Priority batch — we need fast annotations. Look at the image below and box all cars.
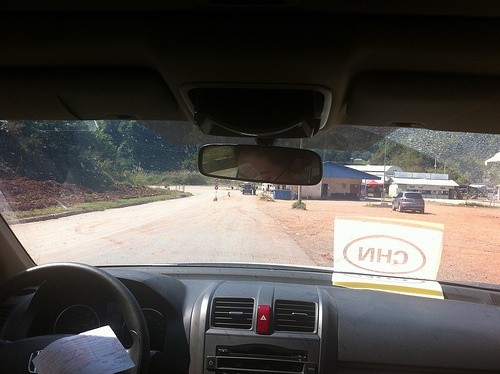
[391,191,424,214]
[242,185,252,195]
